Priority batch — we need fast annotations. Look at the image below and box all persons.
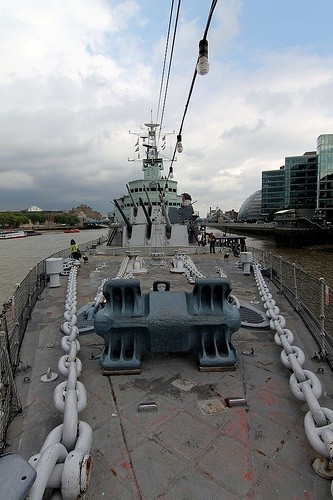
[197,233,216,254]
[70,238,80,261]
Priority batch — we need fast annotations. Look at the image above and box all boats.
[64,229,80,233]
[23,230,42,237]
[0,109,333,500]
[0,231,27,239]
